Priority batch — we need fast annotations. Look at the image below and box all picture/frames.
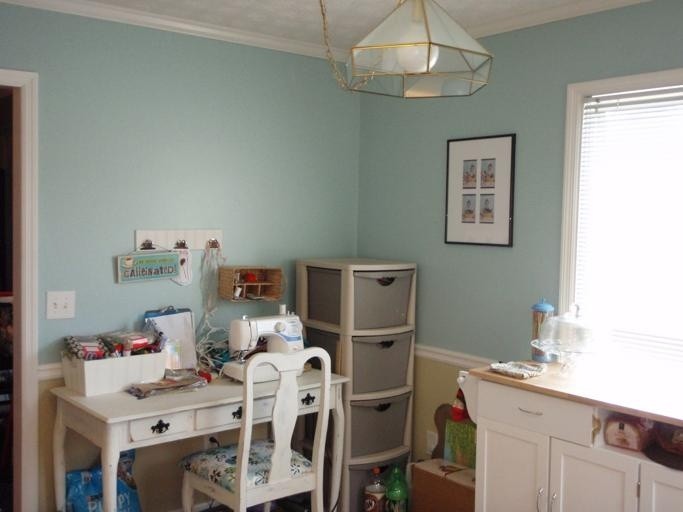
[444,133,518,247]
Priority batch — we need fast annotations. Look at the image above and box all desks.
[50,375,352,510]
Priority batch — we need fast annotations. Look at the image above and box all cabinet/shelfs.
[218,264,282,302]
[293,253,419,510]
[469,358,682,512]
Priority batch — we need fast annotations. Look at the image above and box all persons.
[464,164,476,183]
[481,199,492,217]
[483,163,494,184]
[463,200,475,218]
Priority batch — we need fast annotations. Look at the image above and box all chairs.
[178,345,333,512]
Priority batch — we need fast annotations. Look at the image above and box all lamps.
[339,0,495,100]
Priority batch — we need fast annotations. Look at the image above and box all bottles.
[362,467,386,511]
[386,467,409,511]
[530,297,554,362]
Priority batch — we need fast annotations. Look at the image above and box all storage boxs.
[60,348,165,397]
